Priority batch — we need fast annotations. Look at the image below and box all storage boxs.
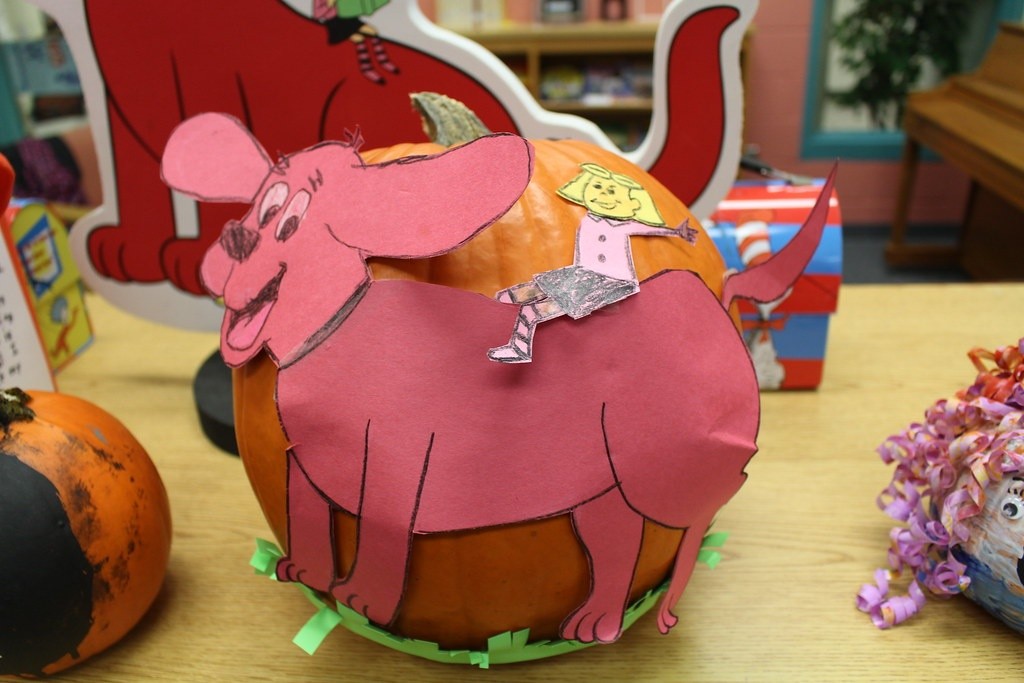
[0,191,54,394]
[702,178,842,393]
[11,198,95,374]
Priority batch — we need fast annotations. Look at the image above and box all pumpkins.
[233,90,744,652]
[0,387,173,679]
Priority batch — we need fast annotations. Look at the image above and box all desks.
[0,282,1024,682]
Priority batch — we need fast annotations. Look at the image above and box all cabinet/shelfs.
[467,27,753,155]
